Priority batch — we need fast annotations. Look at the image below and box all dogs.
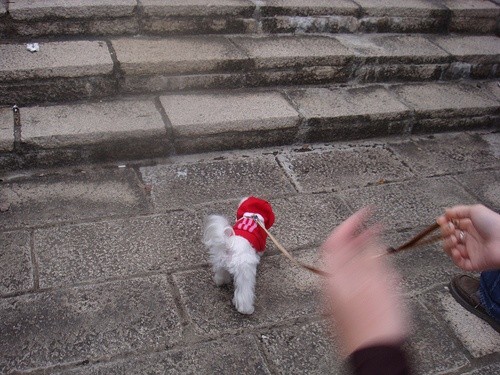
[201,196,275,315]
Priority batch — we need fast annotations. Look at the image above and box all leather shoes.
[449,273,500,335]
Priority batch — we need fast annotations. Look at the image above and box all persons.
[321,203,500,375]
[449,269,500,334]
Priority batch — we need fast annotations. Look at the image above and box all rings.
[459,229,465,243]
